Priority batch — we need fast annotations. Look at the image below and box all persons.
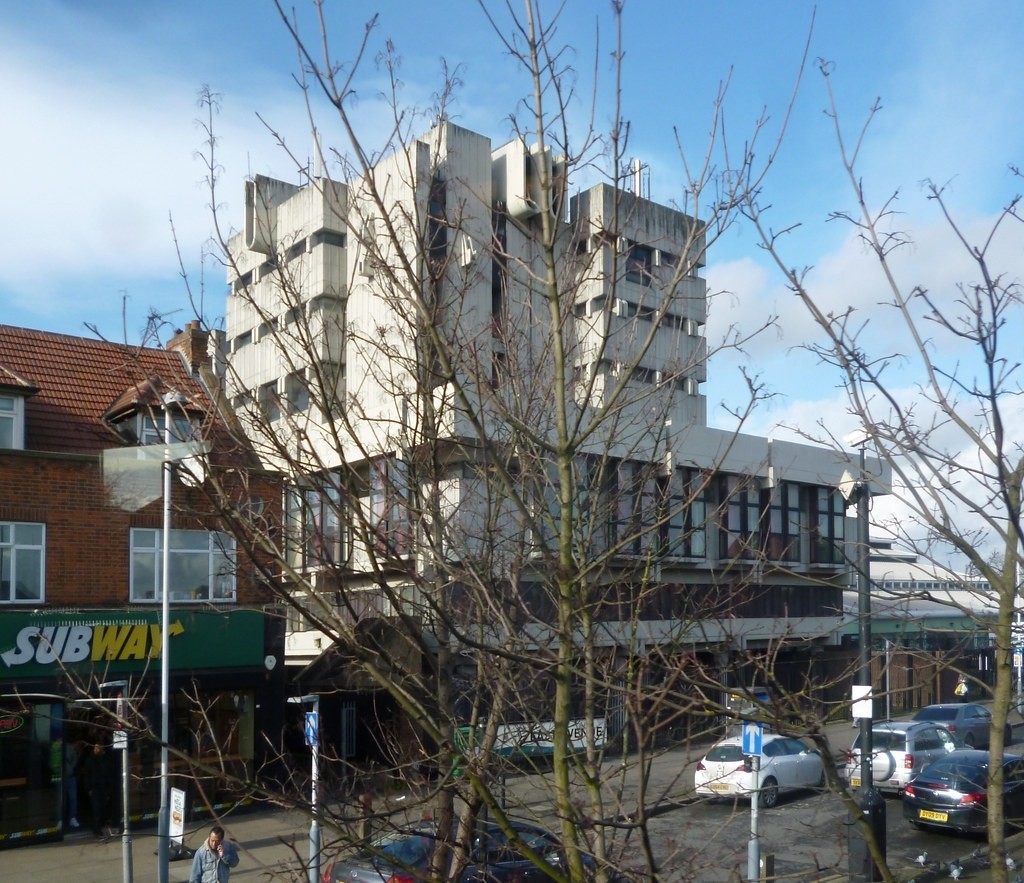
[189,826,239,883]
[957,673,968,703]
[80,741,119,841]
[49,729,79,830]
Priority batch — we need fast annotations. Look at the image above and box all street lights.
[288,693,322,883]
[97,680,139,883]
[155,391,187,883]
[843,429,891,883]
[728,686,773,883]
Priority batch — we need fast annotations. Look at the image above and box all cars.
[912,703,1013,749]
[323,813,598,883]
[902,748,1024,835]
[693,733,832,810]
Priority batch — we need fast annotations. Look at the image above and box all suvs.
[844,719,976,796]
